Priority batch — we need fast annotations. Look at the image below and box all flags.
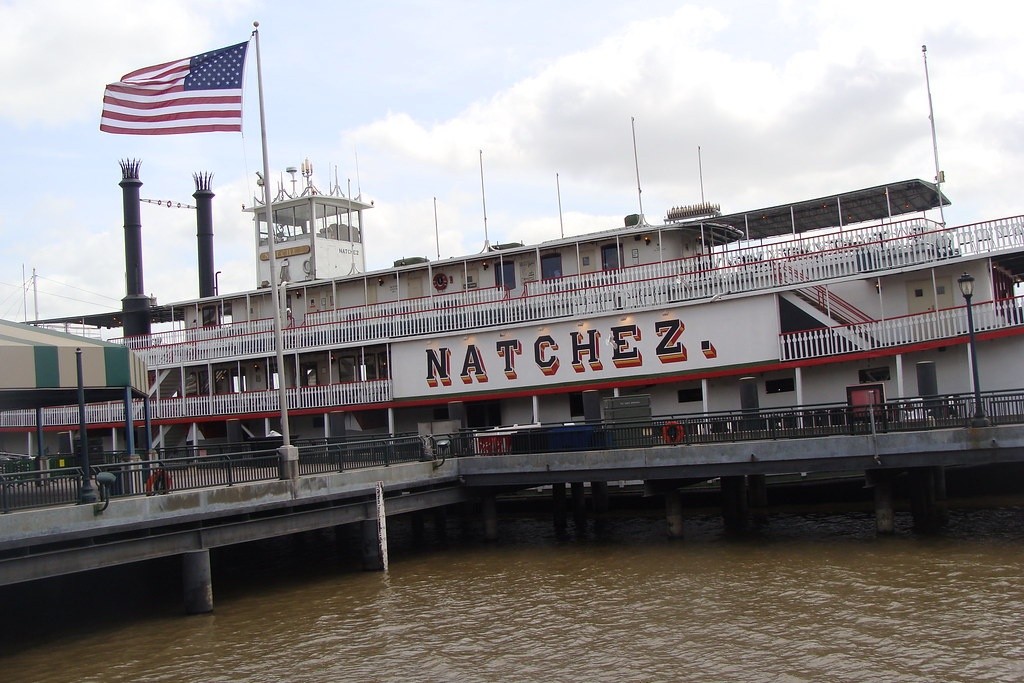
[99,40,250,136]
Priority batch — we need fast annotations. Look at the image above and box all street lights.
[957,272,990,428]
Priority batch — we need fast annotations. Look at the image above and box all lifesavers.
[433,273,449,291]
[147,472,173,496]
[663,420,685,446]
